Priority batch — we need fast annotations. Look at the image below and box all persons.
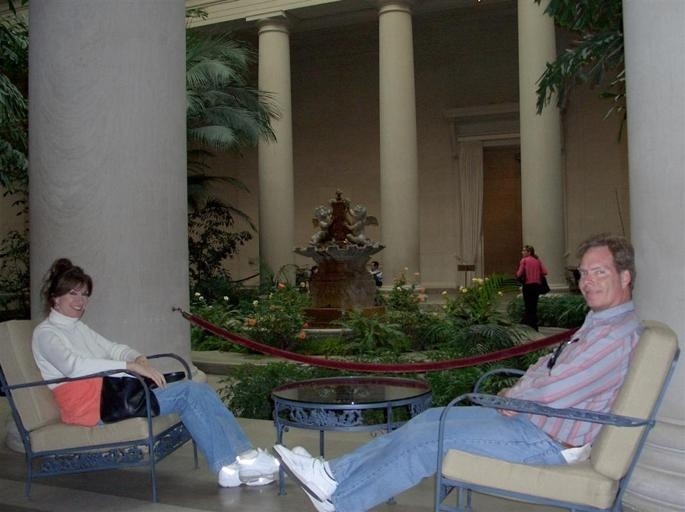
[271,231,640,511]
[29,257,288,488]
[369,260,383,287]
[513,244,548,331]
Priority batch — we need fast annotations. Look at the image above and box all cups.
[547,336,579,376]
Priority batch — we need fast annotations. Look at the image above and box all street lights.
[100,371,186,423]
[538,276,550,295]
[516,259,527,284]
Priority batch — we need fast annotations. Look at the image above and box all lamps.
[1,318,199,504]
[435,321,680,512]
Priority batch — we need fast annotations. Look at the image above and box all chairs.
[291,446,337,512]
[217,459,276,488]
[272,443,339,502]
[235,447,289,484]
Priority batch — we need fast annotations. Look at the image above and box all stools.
[544,432,573,448]
